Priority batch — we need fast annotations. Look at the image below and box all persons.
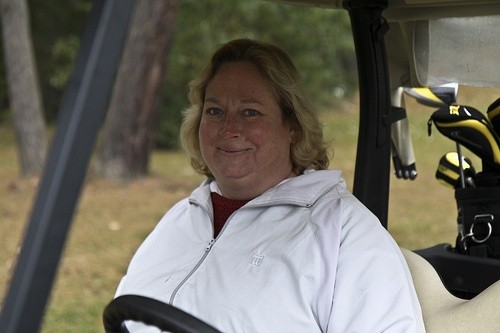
[110,39,429,333]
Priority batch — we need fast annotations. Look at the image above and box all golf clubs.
[439,82,468,188]
